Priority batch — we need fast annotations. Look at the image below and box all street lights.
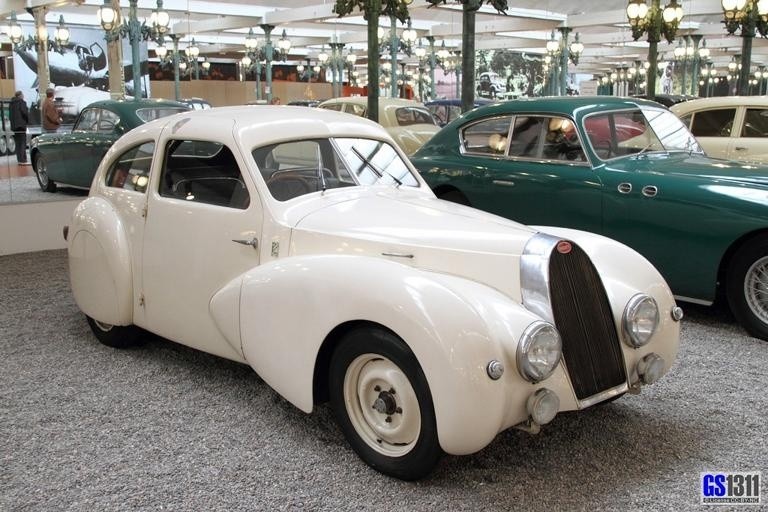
[8,9,69,106]
[184,37,199,79]
[245,0,767,105]
[97,0,169,98]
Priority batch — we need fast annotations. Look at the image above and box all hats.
[46,88,54,93]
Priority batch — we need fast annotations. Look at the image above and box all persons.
[40,88,63,134]
[8,91,31,165]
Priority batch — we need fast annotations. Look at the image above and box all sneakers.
[17,161,31,165]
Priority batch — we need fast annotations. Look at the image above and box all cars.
[63,103,685,482]
[424,99,494,128]
[563,92,700,149]
[616,93,767,165]
[407,94,767,342]
[315,95,443,158]
[30,98,193,192]
